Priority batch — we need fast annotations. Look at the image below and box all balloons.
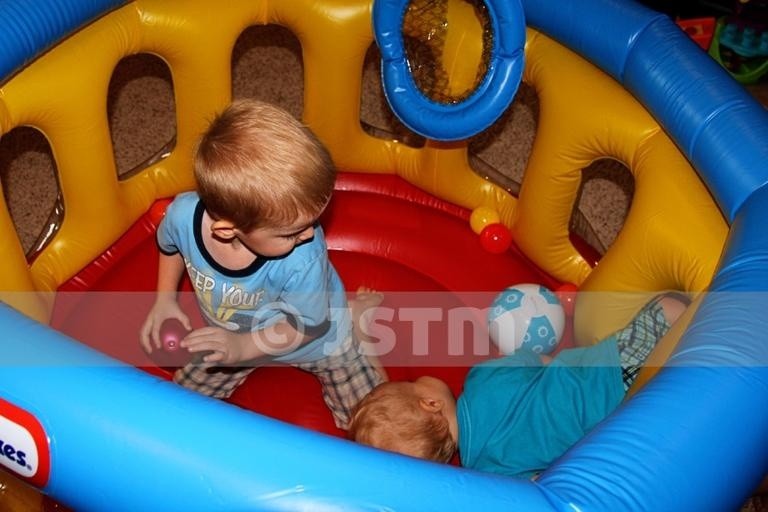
[477,222,512,255]
[469,204,500,235]
[144,319,196,369]
[555,282,579,316]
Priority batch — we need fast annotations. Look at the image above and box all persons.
[344,290,690,482]
[138,98,392,432]
